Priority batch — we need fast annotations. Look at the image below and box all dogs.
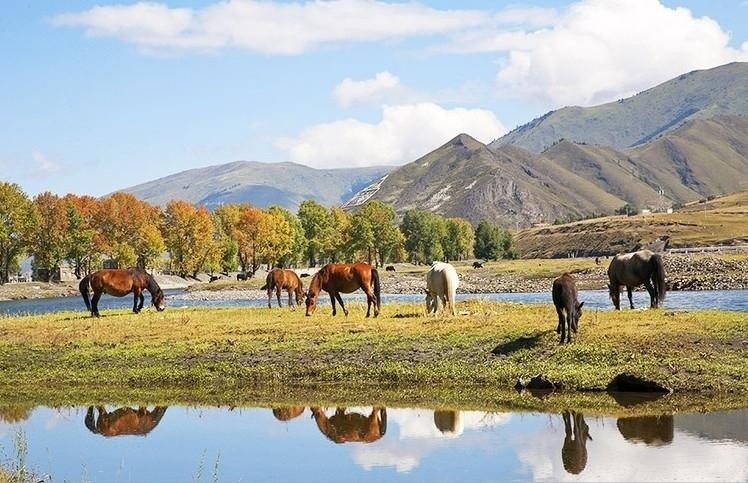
[561,410,594,475]
[305,262,381,318]
[78,266,165,318]
[606,249,667,311]
[552,272,584,345]
[259,269,306,310]
[423,261,459,316]
[309,405,387,445]
[84,405,169,438]
